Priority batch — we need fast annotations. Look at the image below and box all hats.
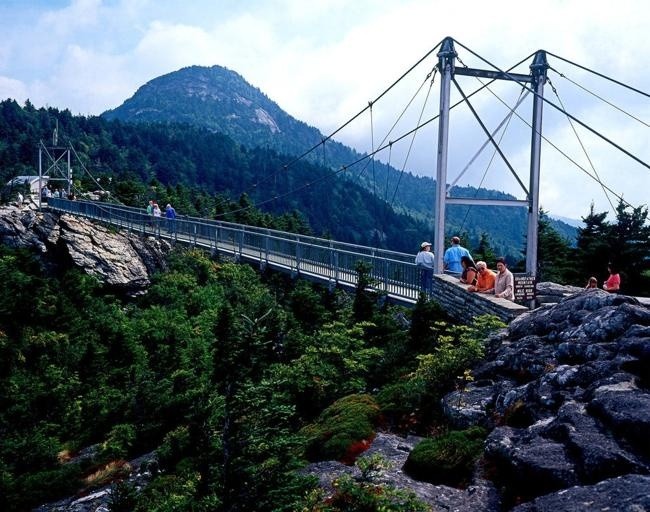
[421,241,432,248]
[451,236,460,243]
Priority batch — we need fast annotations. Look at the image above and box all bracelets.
[474,288,477,291]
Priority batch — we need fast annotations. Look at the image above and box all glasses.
[478,267,485,271]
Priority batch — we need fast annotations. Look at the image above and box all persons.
[596,260,621,293]
[414,242,434,299]
[459,256,479,287]
[616,270,632,295]
[16,191,24,209]
[147,200,161,235]
[443,237,476,280]
[467,260,496,292]
[165,204,178,234]
[480,259,515,302]
[583,275,598,289]
[42,184,77,201]
